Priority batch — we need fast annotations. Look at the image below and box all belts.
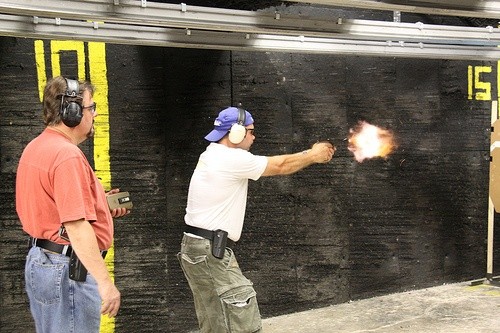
[33,238,107,261]
[184,224,235,251]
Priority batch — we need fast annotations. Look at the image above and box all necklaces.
[49,125,73,141]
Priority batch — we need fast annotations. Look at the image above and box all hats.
[204,107,254,142]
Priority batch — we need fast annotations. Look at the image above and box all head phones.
[61,79,83,127]
[229,107,247,145]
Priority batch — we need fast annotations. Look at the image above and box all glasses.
[83,102,96,112]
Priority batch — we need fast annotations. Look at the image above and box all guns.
[330,137,351,148]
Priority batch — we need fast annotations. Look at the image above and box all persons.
[16,75,132,333]
[177,107,338,333]
